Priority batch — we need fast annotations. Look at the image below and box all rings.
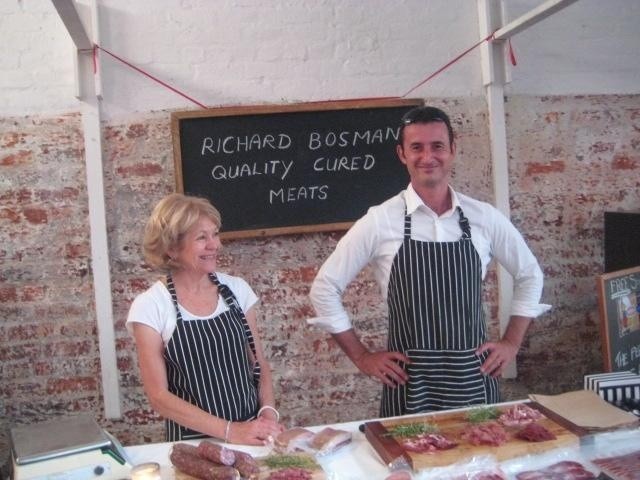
[268,435,274,443]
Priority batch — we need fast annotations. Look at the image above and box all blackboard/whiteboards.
[171,99,424,240]
[597,266,640,376]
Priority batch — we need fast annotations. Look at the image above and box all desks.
[122,388,639,479]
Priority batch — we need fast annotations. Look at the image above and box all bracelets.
[258,405,279,423]
[224,419,231,444]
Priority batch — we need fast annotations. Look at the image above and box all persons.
[308,107,544,419]
[126,193,288,446]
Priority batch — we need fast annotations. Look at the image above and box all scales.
[10,413,134,480]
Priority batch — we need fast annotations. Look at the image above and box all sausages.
[170,441,255,480]
[517,450,640,480]
